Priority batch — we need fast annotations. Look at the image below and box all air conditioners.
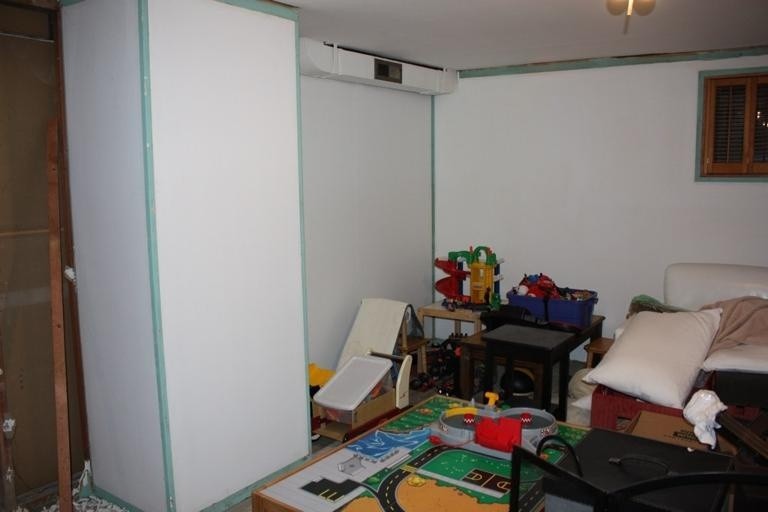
[299,37,458,96]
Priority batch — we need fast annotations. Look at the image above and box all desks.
[251,394,590,512]
[417,301,489,379]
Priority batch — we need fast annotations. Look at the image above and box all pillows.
[581,307,723,412]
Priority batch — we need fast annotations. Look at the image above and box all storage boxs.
[312,355,394,426]
[545,383,742,512]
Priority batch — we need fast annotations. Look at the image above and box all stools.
[480,323,575,422]
[583,337,614,368]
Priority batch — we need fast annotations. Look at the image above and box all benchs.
[614,262,768,397]
